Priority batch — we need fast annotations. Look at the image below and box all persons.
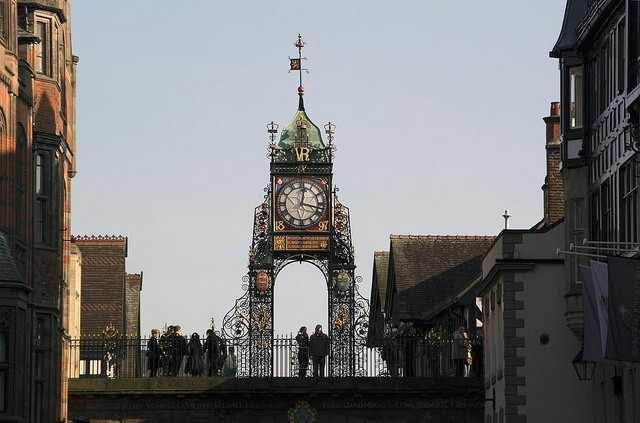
[463,328,473,377]
[309,324,331,377]
[145,325,239,378]
[452,326,465,375]
[297,326,310,376]
